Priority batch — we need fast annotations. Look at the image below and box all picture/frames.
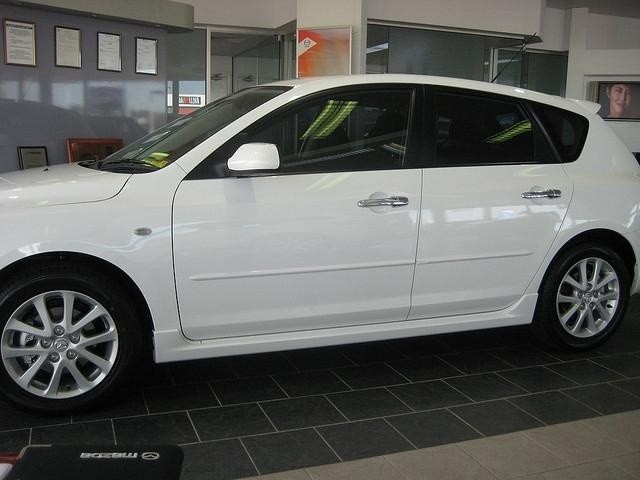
[17,138,124,170]
[3,17,159,76]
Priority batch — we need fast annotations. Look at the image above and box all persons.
[602,84,640,119]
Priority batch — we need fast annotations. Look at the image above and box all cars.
[0,74,640,413]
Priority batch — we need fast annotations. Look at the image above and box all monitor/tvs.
[594,80,640,122]
